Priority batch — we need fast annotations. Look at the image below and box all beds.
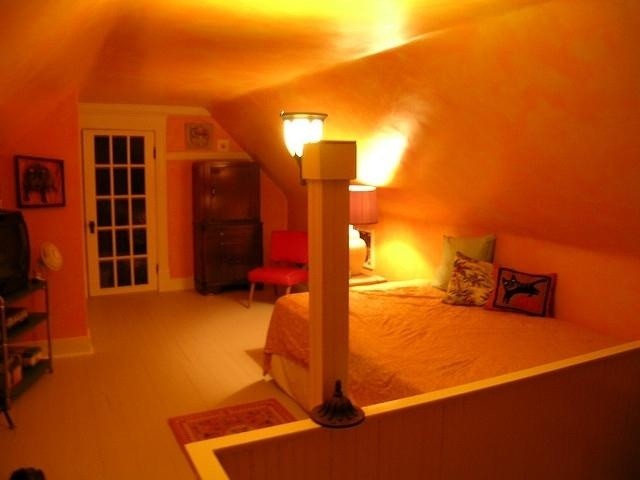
[264,231,640,423]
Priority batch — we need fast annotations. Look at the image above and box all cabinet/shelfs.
[0,279,53,421]
[193,158,262,294]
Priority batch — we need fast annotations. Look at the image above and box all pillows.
[432,233,560,317]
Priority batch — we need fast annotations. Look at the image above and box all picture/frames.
[183,121,213,149]
[15,153,66,210]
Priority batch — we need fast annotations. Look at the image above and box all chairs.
[246,229,308,309]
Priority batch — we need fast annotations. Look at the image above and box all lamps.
[279,111,328,187]
[346,185,379,270]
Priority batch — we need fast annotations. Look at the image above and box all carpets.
[168,397,299,480]
